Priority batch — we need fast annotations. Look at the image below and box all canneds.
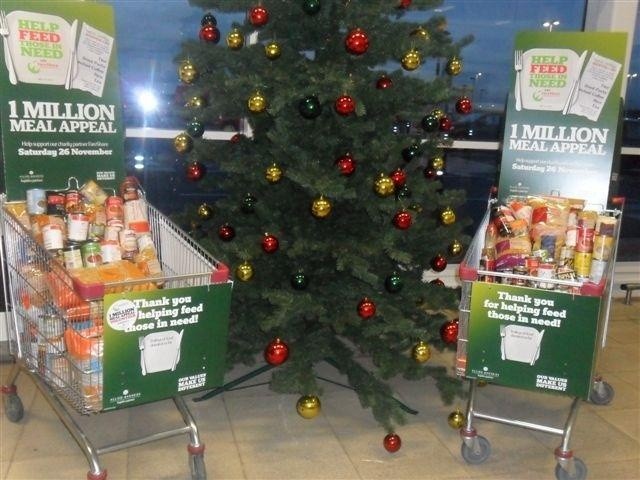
[121,176,138,200]
[79,179,109,206]
[572,214,614,284]
[41,189,101,270]
[119,229,139,261]
[480,259,496,283]
[104,195,124,228]
[26,188,46,214]
[30,314,70,388]
[513,249,555,289]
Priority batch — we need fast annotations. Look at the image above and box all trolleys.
[0,175,234,480]
[455,186,625,480]
